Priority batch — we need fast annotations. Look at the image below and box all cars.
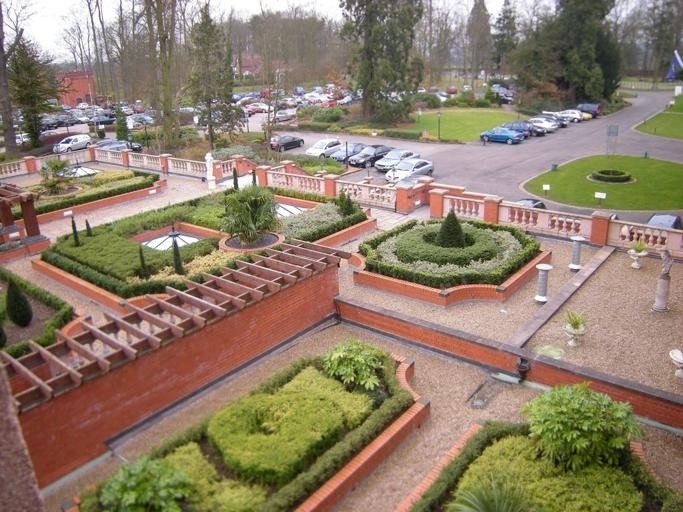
[13,131,58,145]
[0,83,471,130]
[52,133,142,153]
[645,212,681,229]
[305,138,434,182]
[270,135,304,151]
[480,83,601,145]
[513,198,547,210]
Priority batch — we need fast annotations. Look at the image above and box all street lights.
[450,47,461,78]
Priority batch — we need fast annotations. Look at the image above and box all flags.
[665,50,683,82]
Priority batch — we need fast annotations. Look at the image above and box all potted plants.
[626,237,649,270]
[563,309,589,348]
